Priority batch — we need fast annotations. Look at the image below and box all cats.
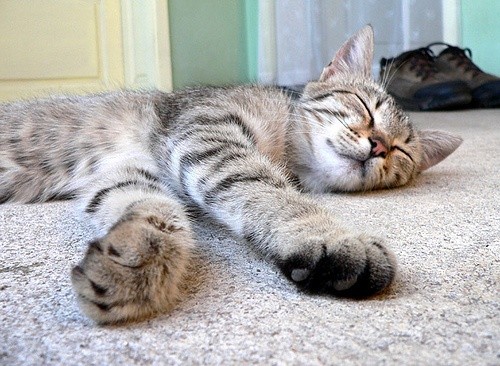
[0,21,465,329]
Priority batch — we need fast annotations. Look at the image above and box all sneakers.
[380,48,473,111]
[424,43,500,108]
